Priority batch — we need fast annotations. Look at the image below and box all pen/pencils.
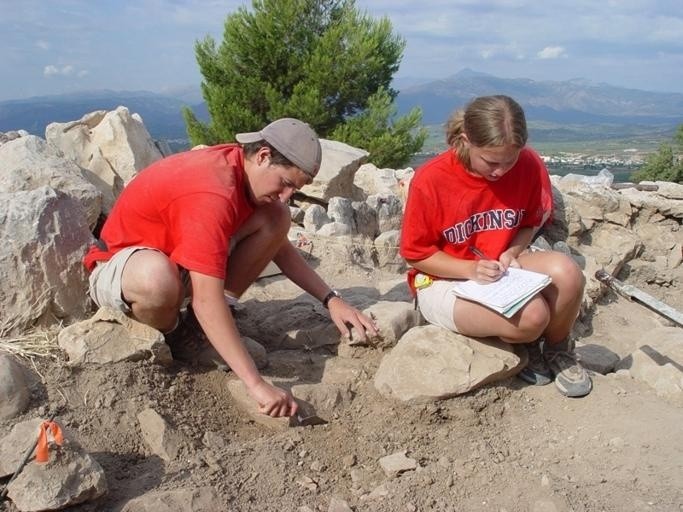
[470,246,488,260]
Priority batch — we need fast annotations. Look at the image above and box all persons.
[79,116,380,418]
[398,92,595,398]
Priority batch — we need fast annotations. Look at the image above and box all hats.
[235,116,322,177]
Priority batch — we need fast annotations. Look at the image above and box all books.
[451,266,553,320]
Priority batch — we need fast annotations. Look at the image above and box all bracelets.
[323,289,342,309]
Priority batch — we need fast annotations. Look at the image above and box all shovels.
[287,409,328,428]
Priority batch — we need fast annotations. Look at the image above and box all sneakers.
[186,302,268,371]
[541,331,593,397]
[516,339,552,387]
[162,310,232,371]
